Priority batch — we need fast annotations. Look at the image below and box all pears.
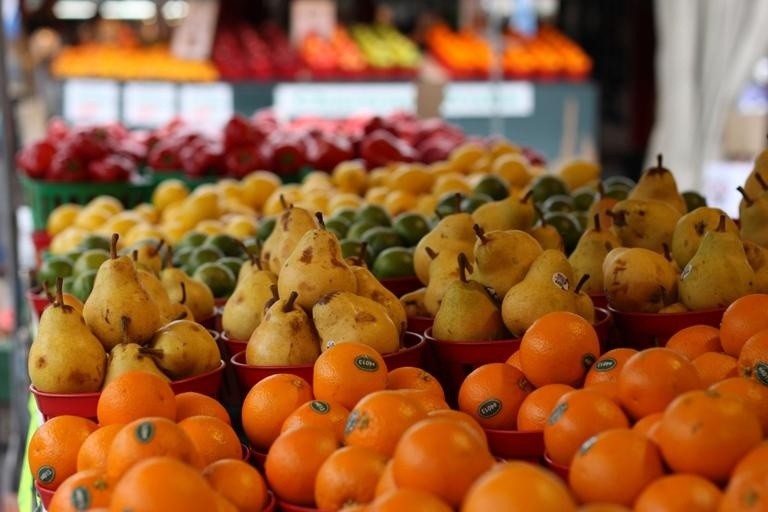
[27,140,768,396]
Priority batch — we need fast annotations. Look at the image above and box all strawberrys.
[16,109,464,183]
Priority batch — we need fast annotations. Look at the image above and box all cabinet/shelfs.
[61,78,600,175]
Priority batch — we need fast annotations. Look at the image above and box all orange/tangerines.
[27,293,768,512]
